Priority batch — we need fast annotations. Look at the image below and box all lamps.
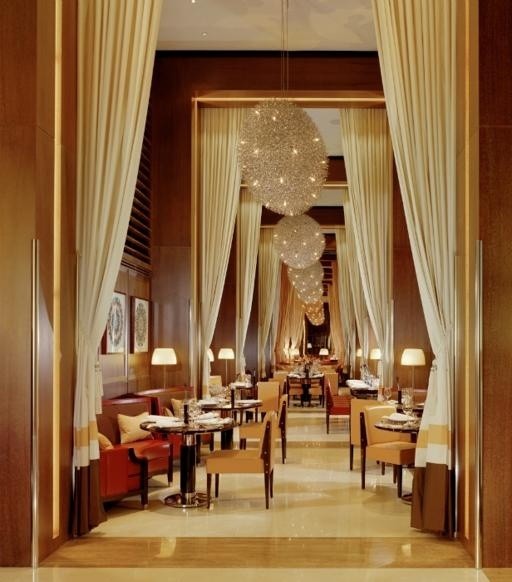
[319,349,329,360]
[218,347,235,388]
[356,349,362,357]
[296,282,324,304]
[303,298,325,326]
[400,348,426,392]
[287,260,324,293]
[370,348,382,379]
[207,348,214,362]
[151,348,177,392]
[234,100,329,217]
[273,215,326,270]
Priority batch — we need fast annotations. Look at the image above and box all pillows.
[164,408,173,417]
[98,432,114,449]
[171,398,183,418]
[117,410,154,443]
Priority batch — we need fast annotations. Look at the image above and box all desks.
[139,417,239,508]
[345,380,378,397]
[286,365,338,373]
[273,371,338,396]
[374,417,420,504]
[396,405,424,413]
[228,382,254,390]
[184,400,263,450]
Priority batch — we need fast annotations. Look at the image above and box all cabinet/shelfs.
[136,387,186,416]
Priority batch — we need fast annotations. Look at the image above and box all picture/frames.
[103,290,126,354]
[132,295,150,354]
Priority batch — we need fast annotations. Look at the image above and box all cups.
[402,401,410,426]
[187,404,201,429]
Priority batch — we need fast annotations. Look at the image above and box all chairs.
[257,381,281,412]
[338,368,344,388]
[349,398,385,476]
[286,375,304,408]
[308,378,324,408]
[239,394,287,464]
[205,410,276,509]
[325,377,358,435]
[360,406,417,498]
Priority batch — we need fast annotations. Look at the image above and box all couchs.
[98,397,173,510]
[134,387,214,467]
